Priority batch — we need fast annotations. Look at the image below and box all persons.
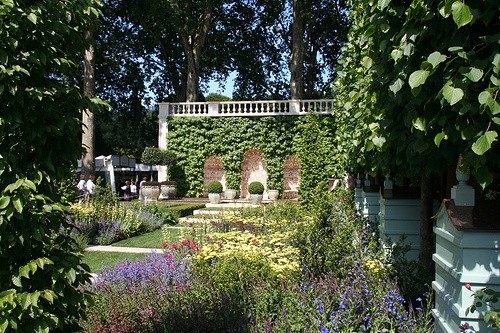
[121,176,147,200]
[77,174,95,196]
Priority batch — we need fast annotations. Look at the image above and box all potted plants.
[138,145,178,202]
[207,179,222,205]
[223,171,240,199]
[248,182,265,204]
[266,183,280,201]
